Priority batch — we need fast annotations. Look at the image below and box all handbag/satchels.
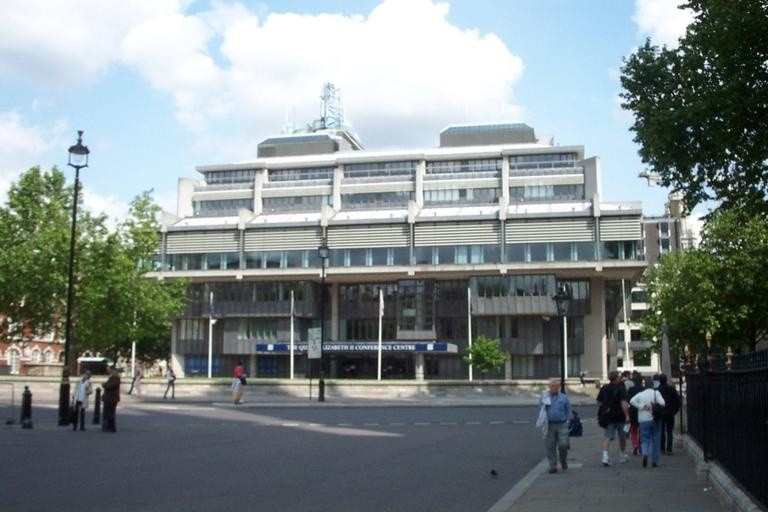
[597,403,614,420]
[653,403,667,420]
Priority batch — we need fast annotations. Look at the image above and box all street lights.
[55,129,95,429]
[131,257,144,379]
[317,238,329,403]
[552,281,570,395]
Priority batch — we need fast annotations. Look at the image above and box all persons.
[102,365,121,433]
[537,369,680,474]
[128,365,141,395]
[71,371,93,432]
[231,360,247,404]
[164,364,175,399]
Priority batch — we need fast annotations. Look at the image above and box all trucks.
[78,356,123,374]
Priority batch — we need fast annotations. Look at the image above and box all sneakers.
[621,455,629,463]
[642,453,657,467]
[603,459,612,466]
